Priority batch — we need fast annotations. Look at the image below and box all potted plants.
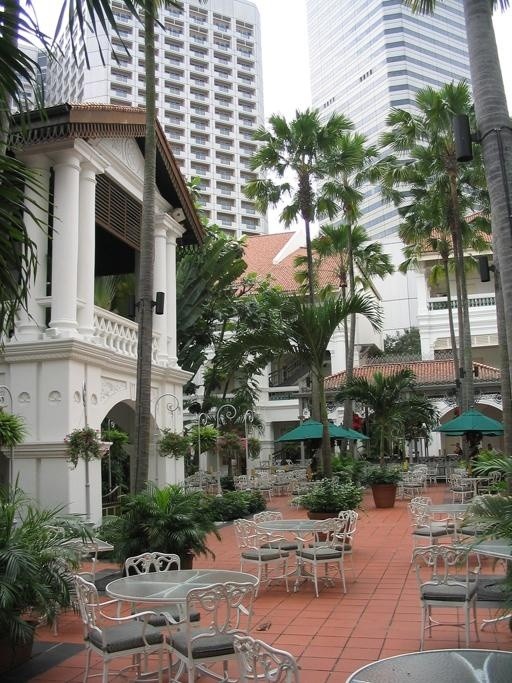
[330,369,442,509]
[93,483,222,569]
[0,472,93,669]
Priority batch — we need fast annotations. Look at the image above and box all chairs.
[213,544,262,627]
[457,523,488,542]
[253,511,301,579]
[413,545,479,647]
[409,496,456,565]
[436,511,456,529]
[310,510,359,576]
[69,573,168,682]
[223,636,301,683]
[230,517,292,594]
[165,583,252,680]
[125,552,200,628]
[182,465,511,506]
[296,518,348,596]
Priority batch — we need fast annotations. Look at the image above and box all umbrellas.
[274,416,353,440]
[432,406,503,431]
[444,433,505,436]
[335,424,371,440]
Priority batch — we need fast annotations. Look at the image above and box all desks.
[457,538,512,629]
[261,519,340,587]
[110,570,258,681]
[346,647,510,682]
[446,516,499,540]
